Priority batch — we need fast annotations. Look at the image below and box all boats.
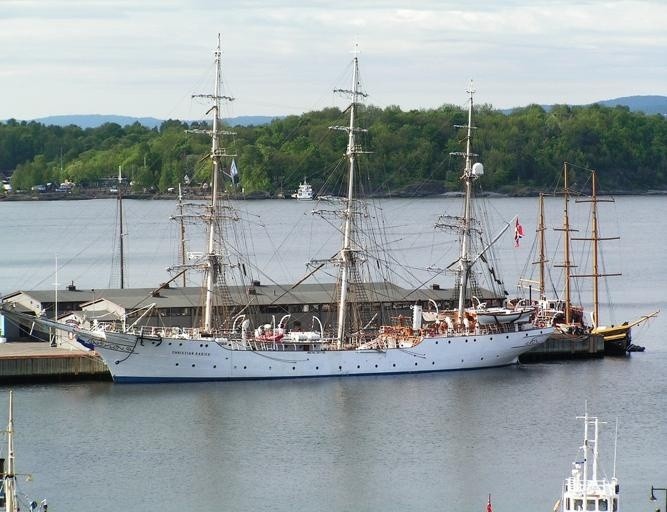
[280,328,328,345]
[421,296,535,324]
[0,390,47,512]
[55,180,76,192]
[290,176,314,201]
[317,195,354,202]
[553,400,620,512]
[31,184,51,193]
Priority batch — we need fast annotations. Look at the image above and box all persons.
[434,315,441,334]
[444,315,471,334]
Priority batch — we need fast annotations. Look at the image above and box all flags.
[516,221,524,246]
[229,158,239,186]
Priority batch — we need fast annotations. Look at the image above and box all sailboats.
[0,32,660,383]
